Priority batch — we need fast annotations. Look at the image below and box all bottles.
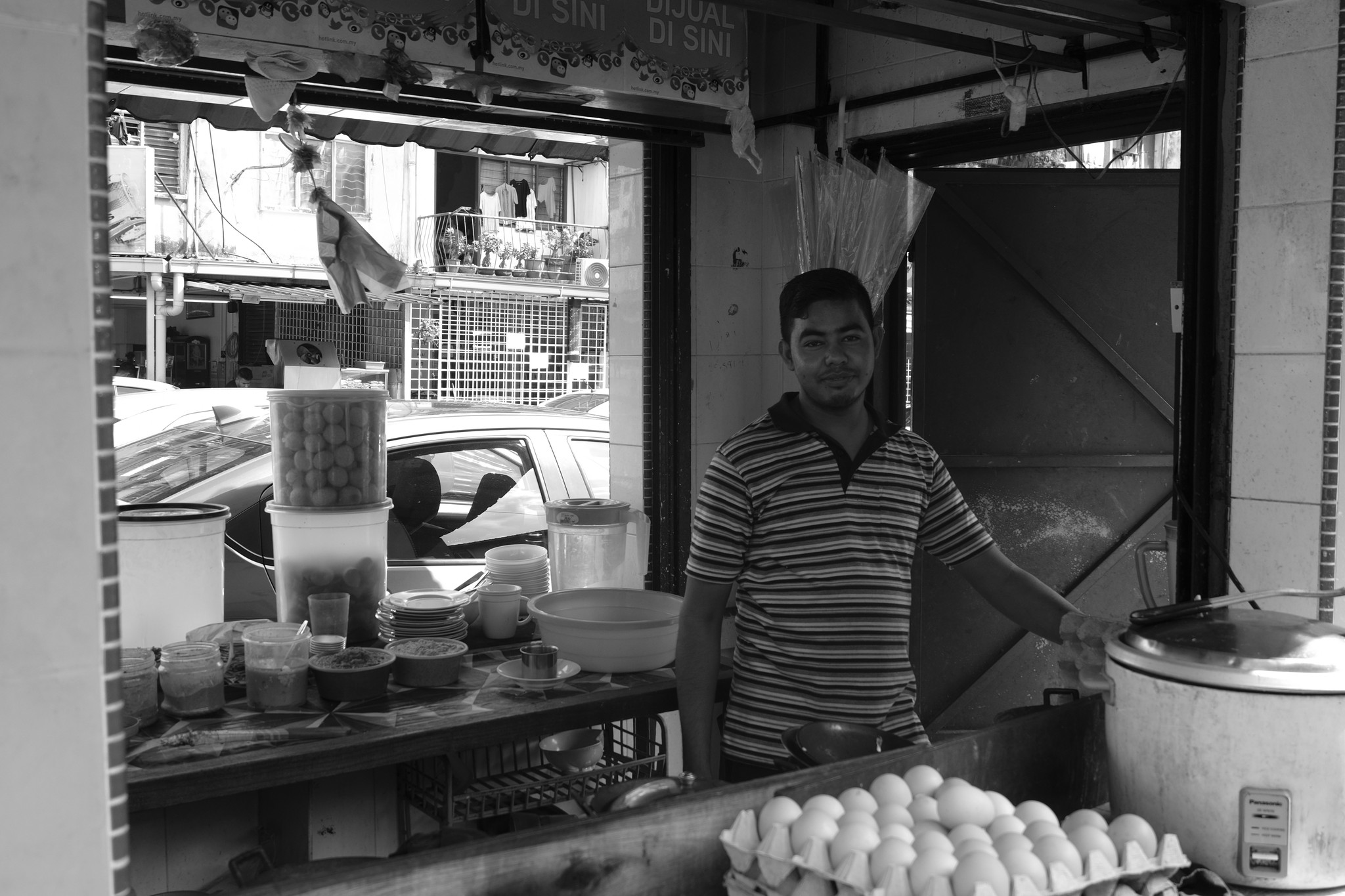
[120,647,158,727]
[158,640,225,717]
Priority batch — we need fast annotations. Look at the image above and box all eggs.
[291,556,379,642]
[756,763,1159,896]
[277,403,385,506]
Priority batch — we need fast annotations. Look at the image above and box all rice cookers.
[1078,607,1345,889]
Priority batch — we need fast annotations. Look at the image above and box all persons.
[118,351,138,378]
[668,266,1085,787]
[225,367,253,388]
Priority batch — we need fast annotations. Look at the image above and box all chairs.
[461,473,528,559]
[391,457,467,559]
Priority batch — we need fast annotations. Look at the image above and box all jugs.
[542,497,650,591]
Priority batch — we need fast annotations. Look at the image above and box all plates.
[375,588,471,643]
[309,635,344,655]
[496,658,581,690]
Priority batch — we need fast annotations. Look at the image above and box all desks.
[122,625,679,896]
[225,692,1345,896]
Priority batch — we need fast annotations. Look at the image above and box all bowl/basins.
[539,729,603,776]
[353,360,386,369]
[241,622,312,710]
[308,637,469,701]
[520,644,559,679]
[484,544,549,616]
[527,587,685,675]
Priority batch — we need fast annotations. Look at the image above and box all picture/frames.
[186,302,215,320]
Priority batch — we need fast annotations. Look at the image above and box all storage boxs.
[132,350,146,366]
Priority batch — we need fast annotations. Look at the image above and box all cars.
[112,374,609,448]
[112,403,610,622]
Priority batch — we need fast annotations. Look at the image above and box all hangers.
[480,177,555,199]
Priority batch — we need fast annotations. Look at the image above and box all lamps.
[228,292,327,305]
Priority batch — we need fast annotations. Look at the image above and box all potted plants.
[410,318,443,391]
[443,227,601,281]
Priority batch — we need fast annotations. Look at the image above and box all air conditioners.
[575,258,610,289]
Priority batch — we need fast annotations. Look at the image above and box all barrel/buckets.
[116,501,231,649]
[263,388,394,654]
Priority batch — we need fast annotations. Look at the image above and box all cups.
[477,584,533,639]
[308,592,350,649]
